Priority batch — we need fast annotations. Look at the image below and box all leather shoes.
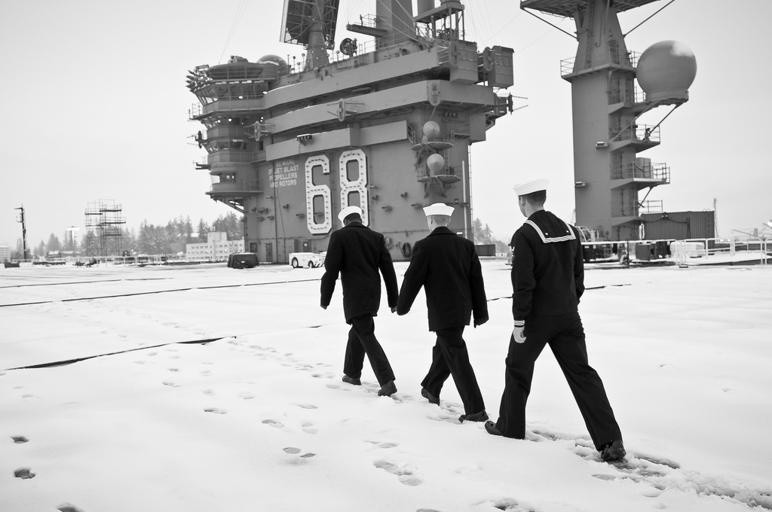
[341,373,626,462]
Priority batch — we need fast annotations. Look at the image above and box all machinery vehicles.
[287,251,328,269]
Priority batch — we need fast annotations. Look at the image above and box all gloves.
[512,320,527,344]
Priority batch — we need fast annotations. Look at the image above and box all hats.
[338,206,362,223]
[511,177,551,197]
[423,202,455,216]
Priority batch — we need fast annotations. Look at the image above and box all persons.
[397,203,490,423]
[486,177,626,461]
[320,205,399,396]
